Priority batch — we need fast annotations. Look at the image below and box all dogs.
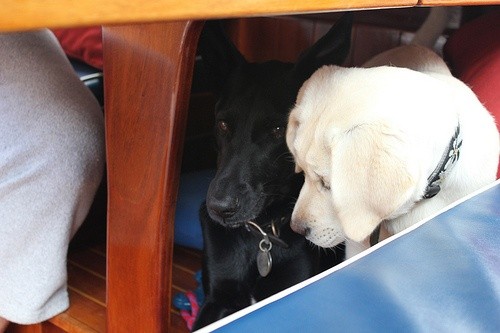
[285,7,500,259]
[192,12,380,331]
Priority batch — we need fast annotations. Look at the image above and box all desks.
[0,0,500,333]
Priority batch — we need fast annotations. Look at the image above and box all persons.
[0,28,105,333]
